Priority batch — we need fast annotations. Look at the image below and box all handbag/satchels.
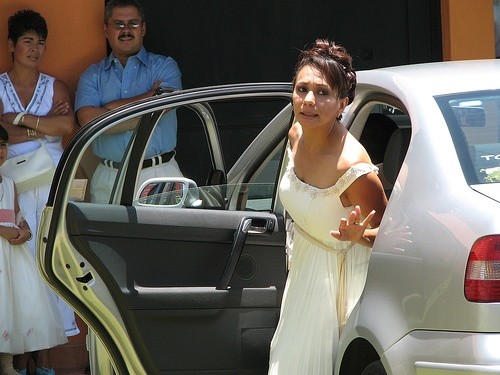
[0,111,56,195]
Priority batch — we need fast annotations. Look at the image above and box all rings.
[13,111,25,126]
[354,220,362,225]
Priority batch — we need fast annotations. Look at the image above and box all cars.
[37,59,500,375]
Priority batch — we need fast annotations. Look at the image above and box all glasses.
[107,21,141,30]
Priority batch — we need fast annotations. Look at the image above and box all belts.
[100,151,174,170]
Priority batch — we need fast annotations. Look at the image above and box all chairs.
[361,112,411,199]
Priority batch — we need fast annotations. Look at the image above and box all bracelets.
[26,128,36,139]
[26,229,33,241]
[34,116,39,132]
[19,113,26,127]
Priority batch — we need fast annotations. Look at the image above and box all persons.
[0,124,70,375]
[72,0,184,205]
[268,39,389,375]
[0,9,80,375]
